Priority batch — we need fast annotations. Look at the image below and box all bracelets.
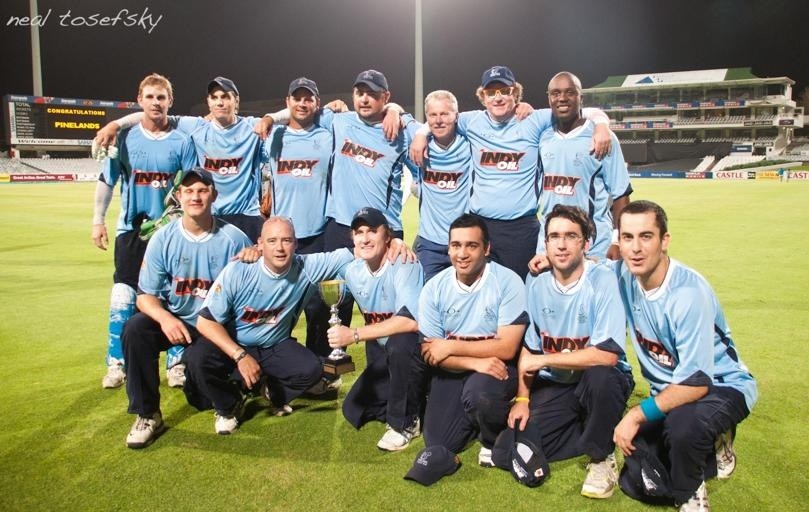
[515,397,529,403]
[235,351,248,363]
[353,328,359,344]
[230,347,243,359]
[640,396,667,425]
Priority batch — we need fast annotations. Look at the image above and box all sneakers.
[103,357,127,388]
[376,416,420,451]
[265,384,293,416]
[215,397,245,435]
[580,452,619,499]
[167,364,186,388]
[126,408,164,448]
[676,425,737,512]
[478,447,496,467]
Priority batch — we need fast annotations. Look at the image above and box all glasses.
[482,86,514,98]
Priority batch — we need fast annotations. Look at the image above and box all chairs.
[1,155,95,183]
[607,96,809,177]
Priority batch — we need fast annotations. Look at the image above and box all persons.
[253,70,420,395]
[422,213,530,467]
[507,204,635,499]
[95,77,350,245]
[91,73,199,389]
[528,200,758,512]
[327,207,434,451]
[182,215,419,434]
[261,77,336,349]
[120,167,261,448]
[385,67,634,283]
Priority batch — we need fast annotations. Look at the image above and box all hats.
[482,66,514,88]
[351,207,387,229]
[509,419,548,487]
[404,445,461,486]
[352,70,388,92]
[628,436,672,497]
[207,76,239,95]
[181,168,214,186]
[288,77,318,95]
